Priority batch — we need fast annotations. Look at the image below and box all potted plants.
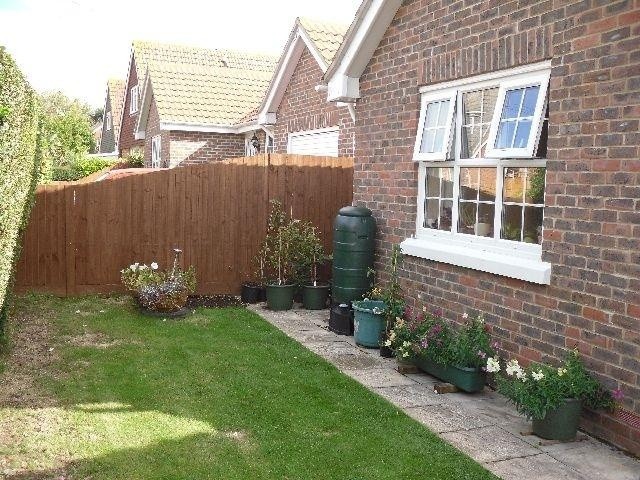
[242,200,329,310]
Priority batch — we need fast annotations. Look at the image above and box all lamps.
[252,132,260,148]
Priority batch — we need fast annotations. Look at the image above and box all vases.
[474,222,491,237]
[532,399,585,439]
[158,292,187,311]
[418,357,485,393]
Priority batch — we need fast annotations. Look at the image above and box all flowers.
[385,306,501,366]
[487,344,615,422]
[120,261,196,311]
